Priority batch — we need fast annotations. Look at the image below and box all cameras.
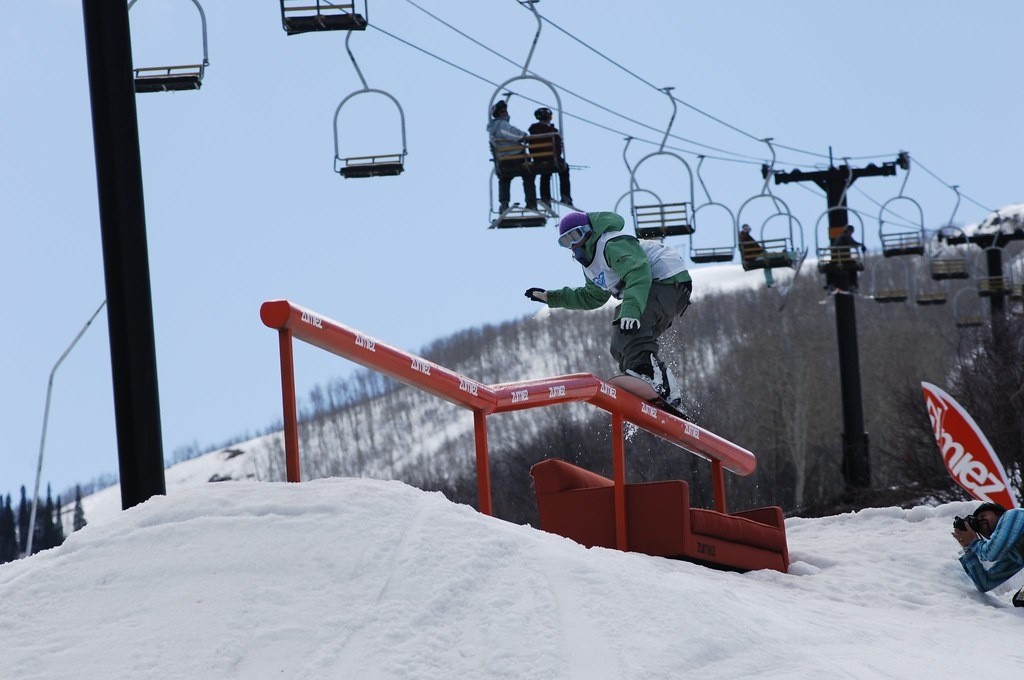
[954,514,982,533]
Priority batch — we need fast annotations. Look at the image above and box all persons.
[487,99,574,210]
[525,211,692,406]
[951,502,1024,607]
[737,224,808,288]
[824,225,866,290]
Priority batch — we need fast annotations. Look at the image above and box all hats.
[973,501,1005,518]
[559,212,589,235]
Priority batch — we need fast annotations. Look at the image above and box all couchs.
[529,460,789,576]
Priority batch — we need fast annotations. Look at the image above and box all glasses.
[557,224,592,249]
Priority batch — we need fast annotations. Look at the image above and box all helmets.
[535,108,552,120]
[492,100,507,118]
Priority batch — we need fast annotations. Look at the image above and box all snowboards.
[608,375,696,423]
[779,247,810,312]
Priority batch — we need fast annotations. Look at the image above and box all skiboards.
[489,203,520,230]
[550,198,584,212]
[518,207,552,218]
[536,198,558,218]
[838,289,872,300]
[818,287,840,305]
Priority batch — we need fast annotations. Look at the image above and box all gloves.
[524,288,548,303]
[611,317,641,336]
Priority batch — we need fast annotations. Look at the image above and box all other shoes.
[560,199,574,207]
[542,198,551,209]
[499,205,509,211]
[525,203,537,210]
